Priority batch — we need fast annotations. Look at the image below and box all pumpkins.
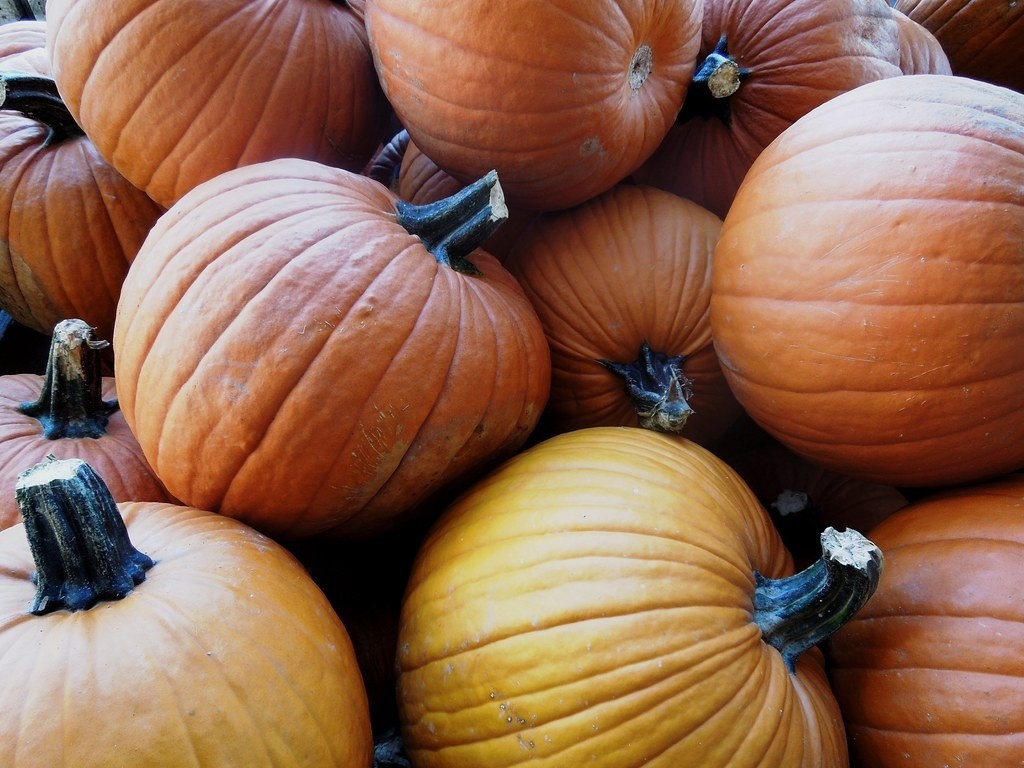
[0,0,1024,768]
